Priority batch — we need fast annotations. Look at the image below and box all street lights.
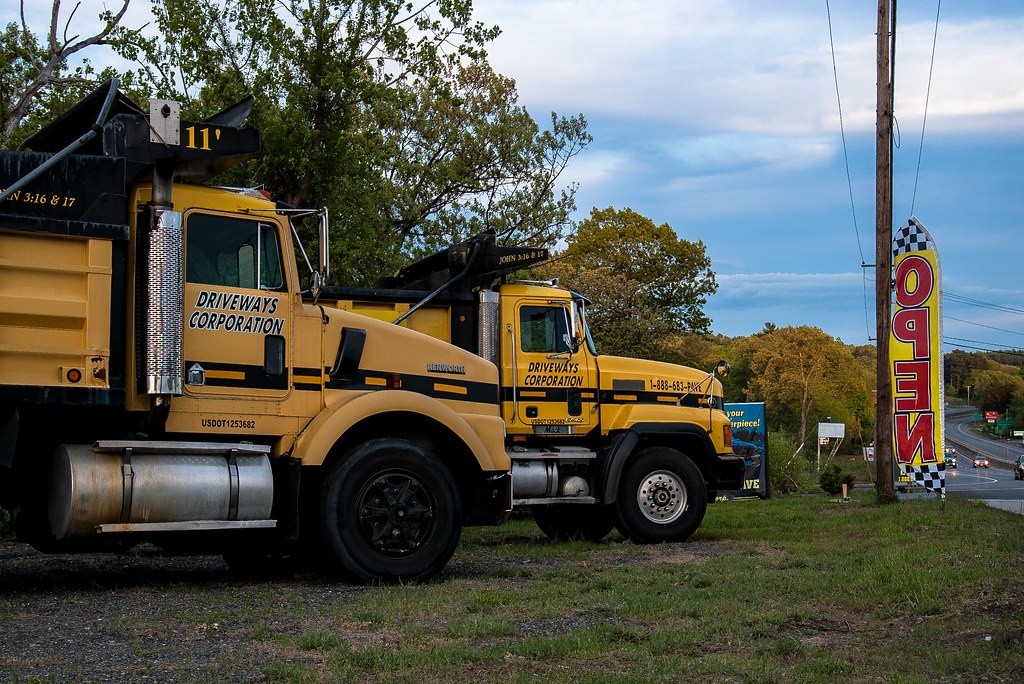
[966,385,971,407]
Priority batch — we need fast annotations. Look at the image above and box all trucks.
[1,76,516,589]
[299,203,748,542]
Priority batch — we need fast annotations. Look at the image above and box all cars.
[1014,455,1024,480]
[944,453,958,469]
[944,445,956,456]
[972,456,990,468]
